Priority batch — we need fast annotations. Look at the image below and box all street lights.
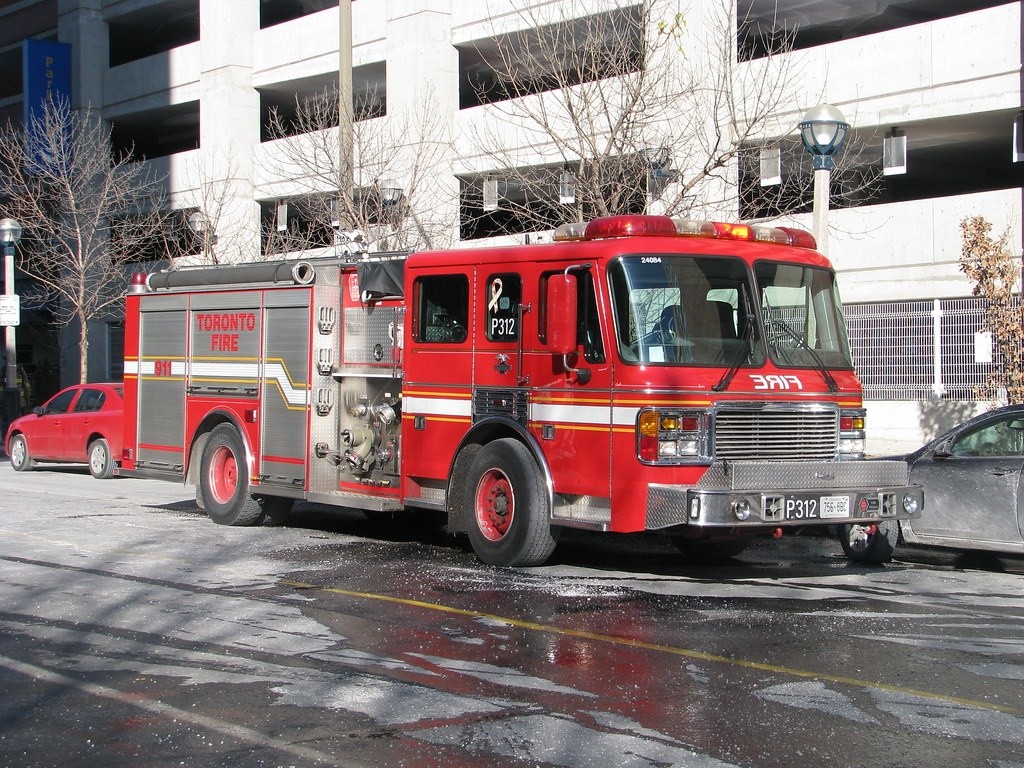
[795,102,852,350]
[0,215,24,429]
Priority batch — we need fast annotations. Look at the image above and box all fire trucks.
[121,212,926,571]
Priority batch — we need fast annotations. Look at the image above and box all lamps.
[639,137,679,183]
[380,178,408,215]
[188,211,218,246]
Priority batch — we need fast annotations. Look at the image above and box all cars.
[836,404,1024,565]
[4,380,122,480]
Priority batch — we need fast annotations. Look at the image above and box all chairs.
[664,301,739,338]
